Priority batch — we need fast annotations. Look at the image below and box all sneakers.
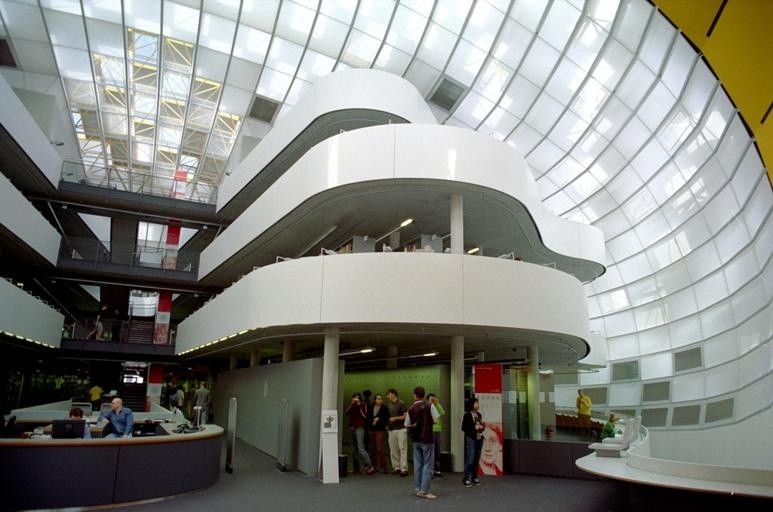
[419,493,436,498]
[472,478,480,483]
[462,479,473,488]
[367,466,408,476]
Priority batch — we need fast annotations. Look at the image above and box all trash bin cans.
[339,455,348,477]
[440,451,451,472]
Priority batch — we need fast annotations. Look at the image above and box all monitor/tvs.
[51,420,85,438]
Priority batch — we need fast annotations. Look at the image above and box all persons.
[344,392,375,475]
[111,309,122,343]
[85,304,109,342]
[477,422,503,476]
[385,389,409,476]
[600,413,621,444]
[404,386,438,499]
[362,390,374,455]
[193,381,211,426]
[89,382,105,411]
[462,398,486,488]
[68,407,92,439]
[427,393,444,478]
[97,397,134,439]
[164,385,187,411]
[365,394,390,475]
[576,389,592,436]
